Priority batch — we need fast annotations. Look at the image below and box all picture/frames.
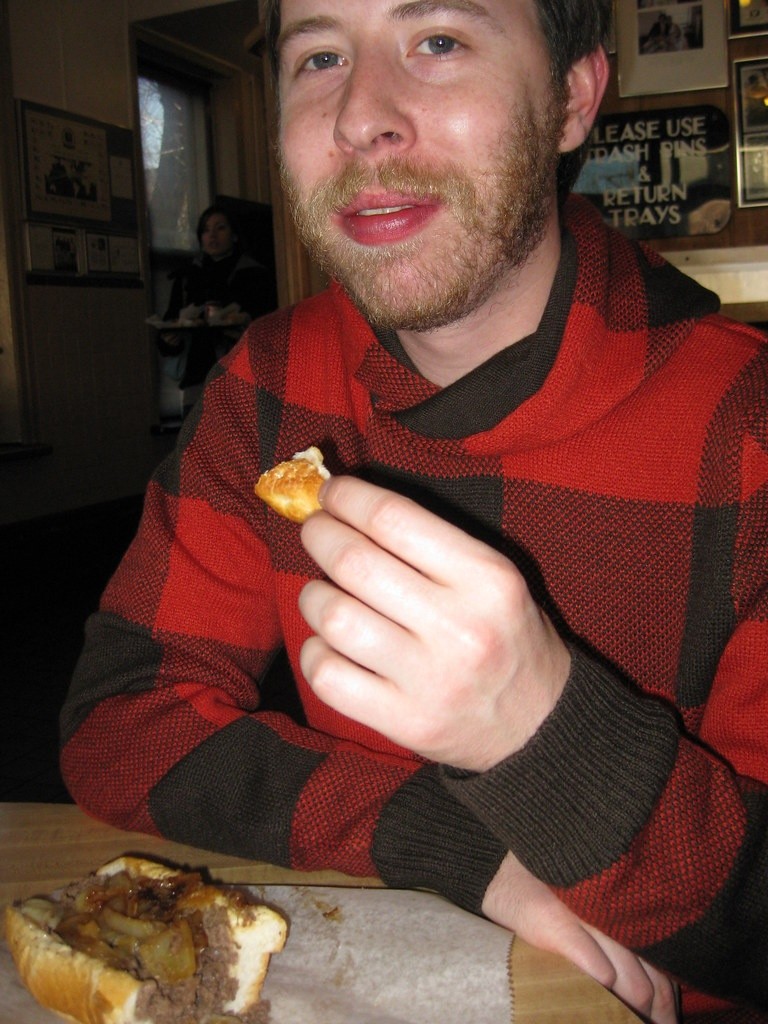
[732,55,768,208]
[614,0,730,97]
[727,0,768,40]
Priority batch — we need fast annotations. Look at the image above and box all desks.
[0,801,645,1024]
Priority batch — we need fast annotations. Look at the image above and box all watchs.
[166,204,279,415]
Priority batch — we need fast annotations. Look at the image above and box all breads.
[252,446,335,524]
[0,857,289,1024]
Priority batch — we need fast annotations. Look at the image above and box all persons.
[64,0,768,1024]
